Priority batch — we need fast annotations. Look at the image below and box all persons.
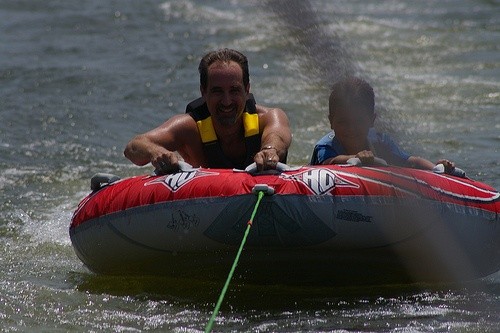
[124,48,292,175]
[308,77,456,175]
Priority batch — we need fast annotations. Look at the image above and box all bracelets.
[260,145,278,153]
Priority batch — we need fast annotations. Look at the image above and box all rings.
[160,161,166,167]
[266,158,273,162]
